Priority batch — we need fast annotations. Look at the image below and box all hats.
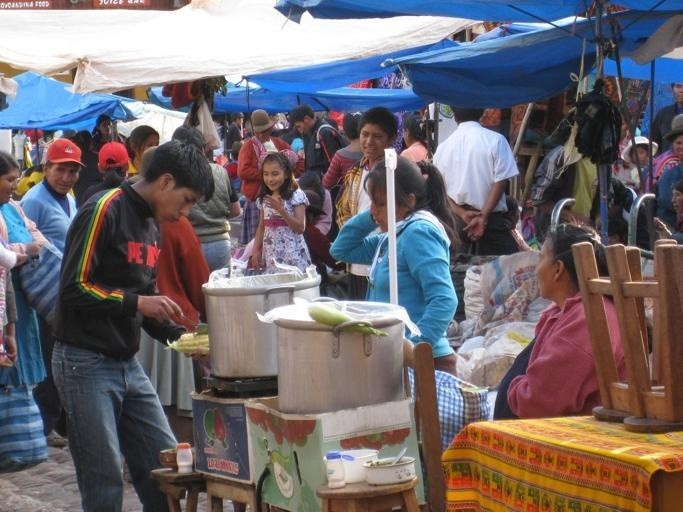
[302,189,327,217]
[45,137,88,168]
[620,135,659,163]
[661,113,683,144]
[98,141,129,170]
[244,108,277,133]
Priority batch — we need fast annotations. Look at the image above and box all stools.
[600,234,683,433]
[202,473,259,512]
[150,465,202,512]
[314,478,421,512]
[563,226,665,422]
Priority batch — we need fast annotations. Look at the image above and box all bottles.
[176,443,193,475]
[324,451,345,489]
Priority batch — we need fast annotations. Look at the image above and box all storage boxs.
[244,396,428,511]
[189,390,253,485]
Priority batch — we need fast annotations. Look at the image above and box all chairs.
[401,339,449,503]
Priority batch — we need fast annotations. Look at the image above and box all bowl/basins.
[322,450,379,484]
[159,447,195,471]
[361,456,416,485]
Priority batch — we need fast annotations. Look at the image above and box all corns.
[163,332,209,353]
[308,302,387,338]
[508,332,532,348]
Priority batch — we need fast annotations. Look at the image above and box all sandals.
[44,429,70,447]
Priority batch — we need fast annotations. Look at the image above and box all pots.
[200,269,322,381]
[270,297,404,414]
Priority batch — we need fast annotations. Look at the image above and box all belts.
[55,335,139,363]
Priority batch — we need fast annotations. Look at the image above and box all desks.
[436,411,680,508]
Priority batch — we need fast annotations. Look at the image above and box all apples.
[340,428,410,450]
[202,408,228,449]
[245,407,316,446]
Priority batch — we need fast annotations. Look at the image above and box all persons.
[213,104,521,385]
[0,114,240,466]
[47,137,219,511]
[495,78,682,419]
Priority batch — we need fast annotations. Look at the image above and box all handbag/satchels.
[384,356,493,505]
[0,365,50,475]
[17,241,65,336]
